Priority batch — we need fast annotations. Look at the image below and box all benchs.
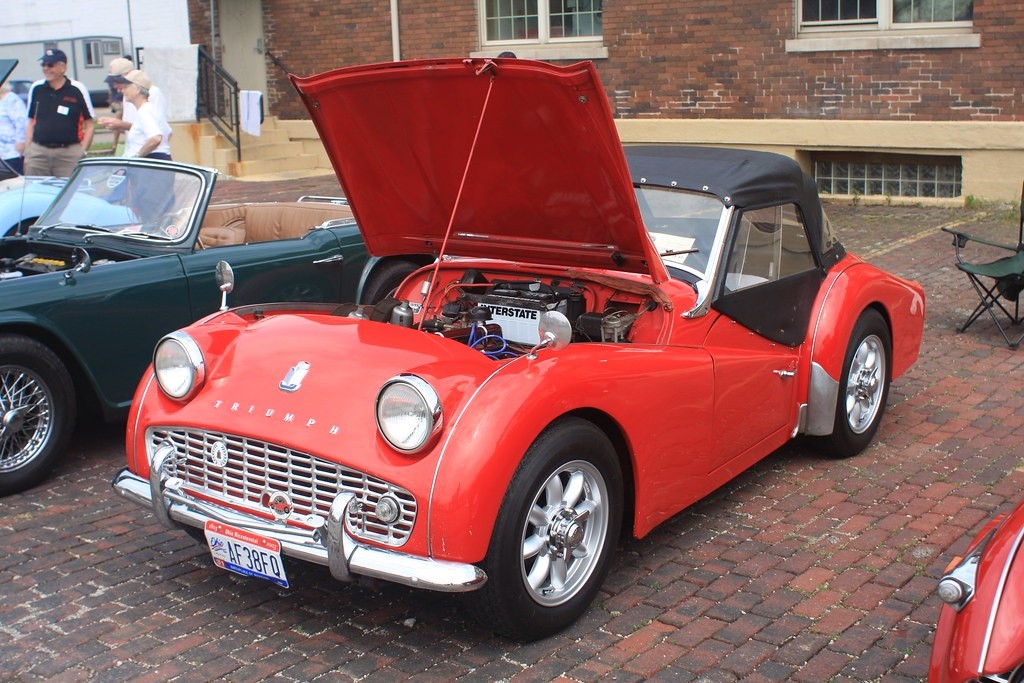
[651,217,815,280]
[176,201,352,242]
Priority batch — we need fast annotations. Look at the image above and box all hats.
[105,56,134,83]
[114,69,151,89]
[39,48,66,63]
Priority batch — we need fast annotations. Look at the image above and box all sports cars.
[0,153,438,497]
[110,55,928,644]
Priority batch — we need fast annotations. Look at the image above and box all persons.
[98,56,174,222]
[22,49,96,177]
[0,80,29,182]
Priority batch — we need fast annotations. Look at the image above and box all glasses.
[41,62,55,68]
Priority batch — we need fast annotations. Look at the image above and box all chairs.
[940,181,1024,352]
[194,227,246,251]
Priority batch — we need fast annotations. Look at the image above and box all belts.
[33,139,78,148]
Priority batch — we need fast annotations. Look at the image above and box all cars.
[927,500,1023,683]
